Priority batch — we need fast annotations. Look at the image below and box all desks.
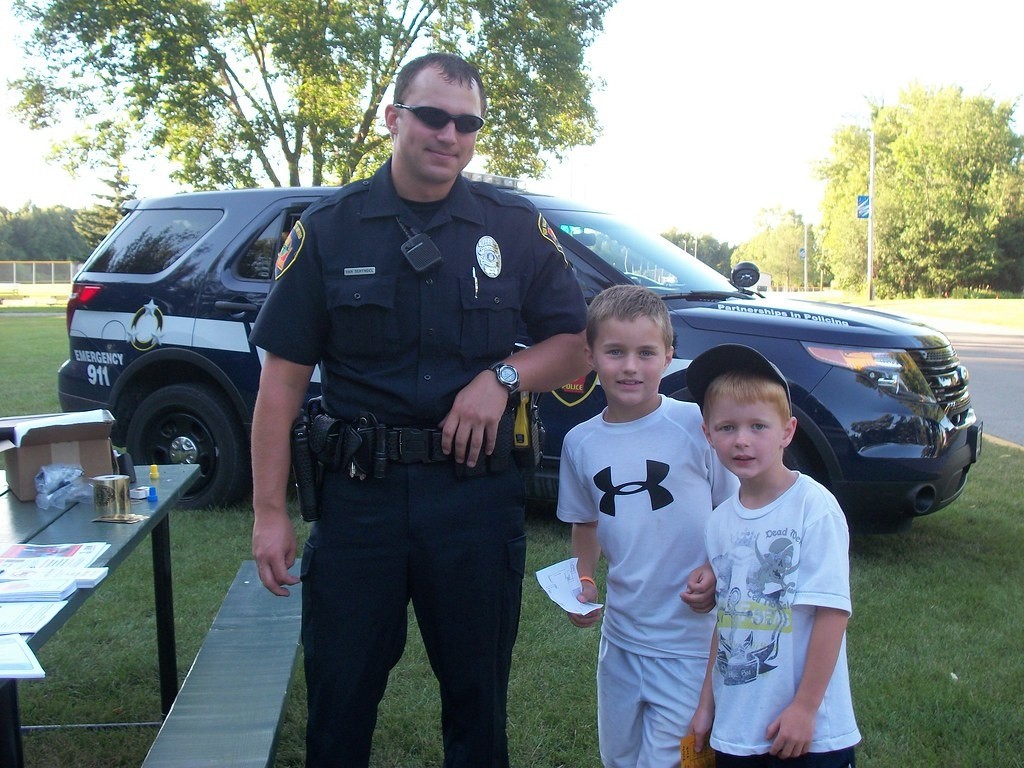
[0,464,201,768]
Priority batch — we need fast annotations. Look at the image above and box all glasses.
[393,103,485,134]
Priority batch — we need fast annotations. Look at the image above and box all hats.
[685,343,792,418]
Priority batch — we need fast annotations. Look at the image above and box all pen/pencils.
[472,265,479,298]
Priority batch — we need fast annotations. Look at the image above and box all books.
[0,542,111,677]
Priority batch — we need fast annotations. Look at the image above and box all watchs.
[486,362,519,396]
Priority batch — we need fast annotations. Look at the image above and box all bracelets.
[580,576,598,603]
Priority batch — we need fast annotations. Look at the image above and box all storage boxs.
[0,409,116,502]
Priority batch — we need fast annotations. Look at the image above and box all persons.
[248,53,584,768]
[557,285,740,768]
[687,342,864,768]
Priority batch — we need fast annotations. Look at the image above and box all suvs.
[57,170,985,542]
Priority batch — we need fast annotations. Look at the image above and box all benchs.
[141,559,303,768]
